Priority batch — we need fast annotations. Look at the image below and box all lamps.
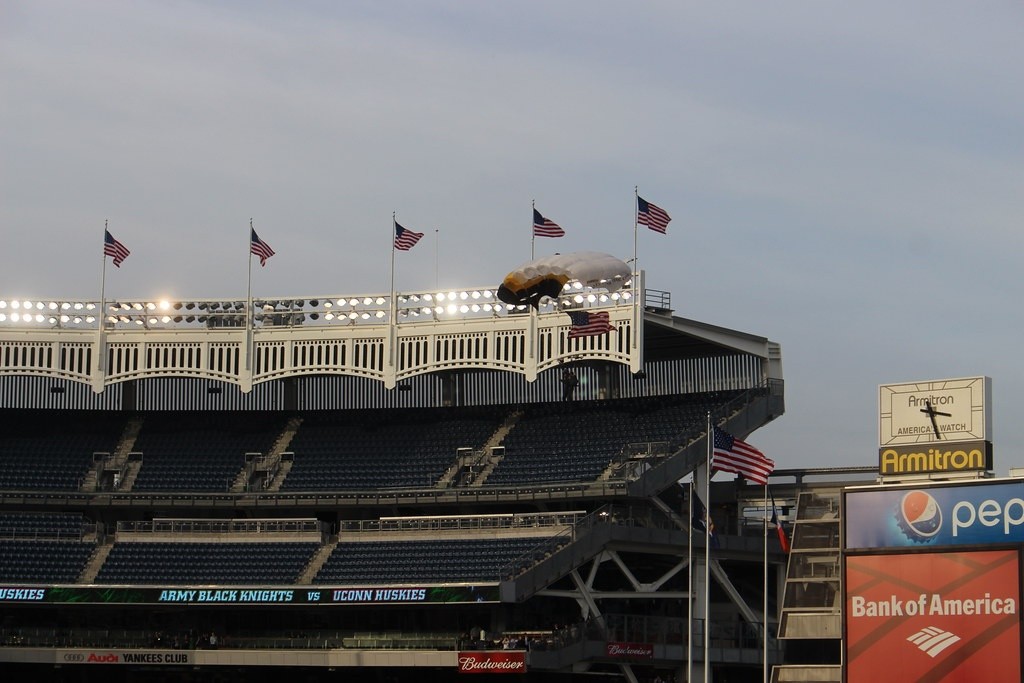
[0,276,631,324]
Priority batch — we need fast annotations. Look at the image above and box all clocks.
[879,376,991,450]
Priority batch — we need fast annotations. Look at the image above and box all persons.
[561,368,580,401]
[3,627,219,650]
[461,622,570,651]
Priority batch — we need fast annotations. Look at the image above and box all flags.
[394,222,425,252]
[637,196,672,234]
[104,230,130,269]
[250,229,275,267]
[565,310,618,338]
[770,506,790,554]
[533,209,565,238]
[710,424,776,486]
